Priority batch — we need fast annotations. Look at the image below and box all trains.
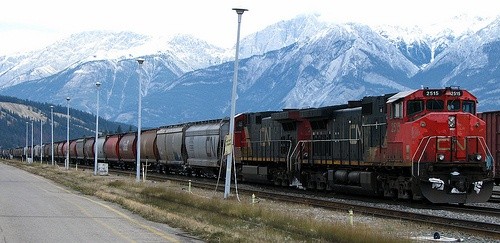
[0,84,496,207]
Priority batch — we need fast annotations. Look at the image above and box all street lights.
[39,113,45,164]
[93,82,102,176]
[26,120,29,161]
[64,96,71,169]
[222,7,250,199]
[29,116,34,161]
[136,57,146,182]
[49,105,54,166]
